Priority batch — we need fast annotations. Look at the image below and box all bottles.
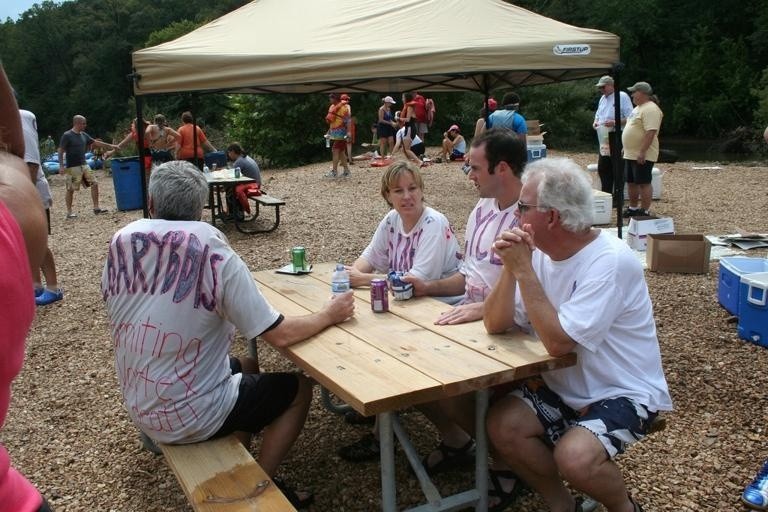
[331,262,352,323]
[325,137,330,148]
[202,163,210,177]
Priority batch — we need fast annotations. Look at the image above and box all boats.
[41,149,102,174]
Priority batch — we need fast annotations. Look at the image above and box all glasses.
[517,200,551,212]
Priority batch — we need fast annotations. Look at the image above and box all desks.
[240,259,576,512]
[201,169,256,226]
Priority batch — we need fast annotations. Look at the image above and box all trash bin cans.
[110,156,144,211]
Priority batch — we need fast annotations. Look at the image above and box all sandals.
[406,438,476,477]
[485,466,524,511]
[32,282,62,304]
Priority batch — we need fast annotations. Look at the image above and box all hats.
[340,94,350,100]
[385,96,396,104]
[595,76,614,87]
[447,125,459,132]
[627,82,652,92]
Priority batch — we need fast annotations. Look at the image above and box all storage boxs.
[643,229,712,273]
[627,215,674,252]
[614,166,660,199]
[720,256,768,320]
[735,271,768,348]
[523,119,547,161]
[589,186,613,226]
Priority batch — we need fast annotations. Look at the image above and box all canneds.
[235,168,240,178]
[344,135,350,143]
[292,247,305,272]
[370,279,388,313]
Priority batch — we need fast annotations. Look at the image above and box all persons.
[99,160,355,512]
[398,127,527,512]
[89,108,218,190]
[623,82,665,218]
[221,141,261,222]
[57,114,121,218]
[592,75,634,208]
[334,160,467,462]
[489,155,675,512]
[0,69,62,511]
[323,86,527,178]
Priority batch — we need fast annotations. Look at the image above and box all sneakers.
[340,171,350,177]
[622,208,650,218]
[93,208,108,213]
[340,431,379,459]
[323,171,337,178]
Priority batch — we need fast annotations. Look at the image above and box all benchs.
[139,418,303,512]
[247,189,286,233]
